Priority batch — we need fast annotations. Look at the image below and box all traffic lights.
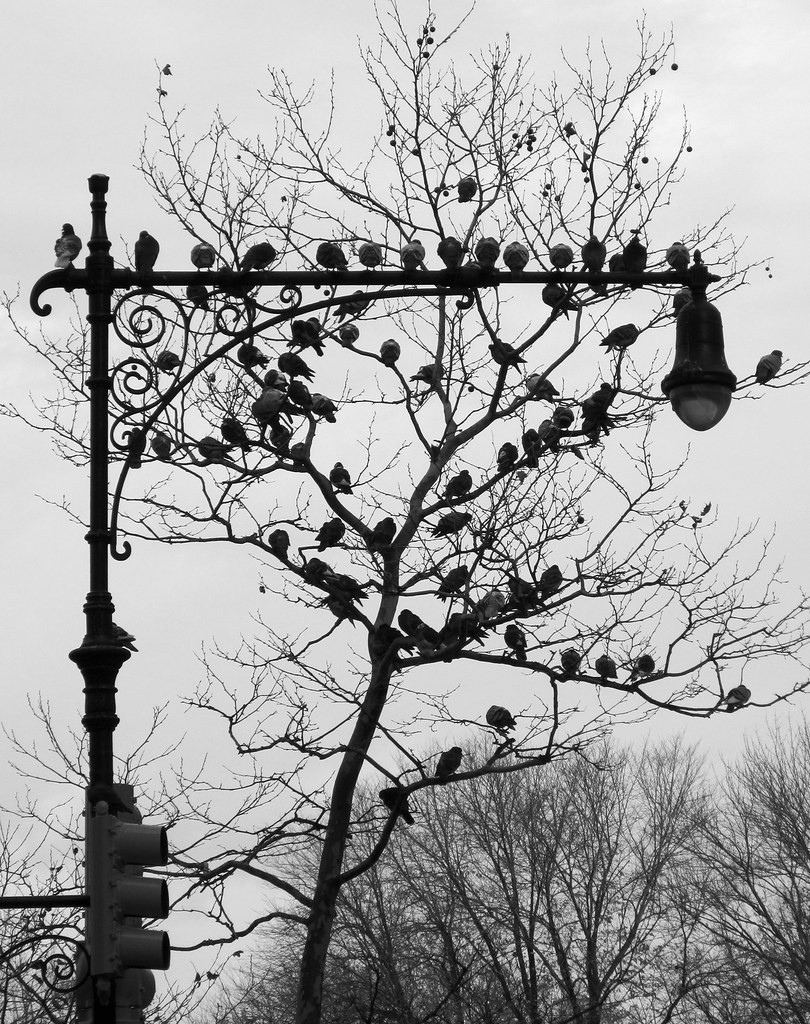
[89,805,168,973]
[132,969,158,1018]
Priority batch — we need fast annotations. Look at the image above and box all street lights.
[23,168,742,1024]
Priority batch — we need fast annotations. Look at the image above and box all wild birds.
[755,347,784,385]
[398,561,752,710]
[52,219,688,541]
[377,703,519,825]
[268,518,397,607]
